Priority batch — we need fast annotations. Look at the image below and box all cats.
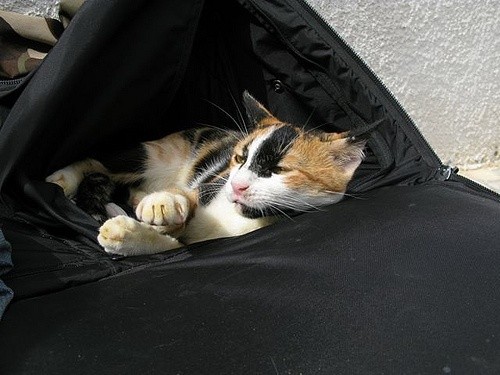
[45,90,385,257]
